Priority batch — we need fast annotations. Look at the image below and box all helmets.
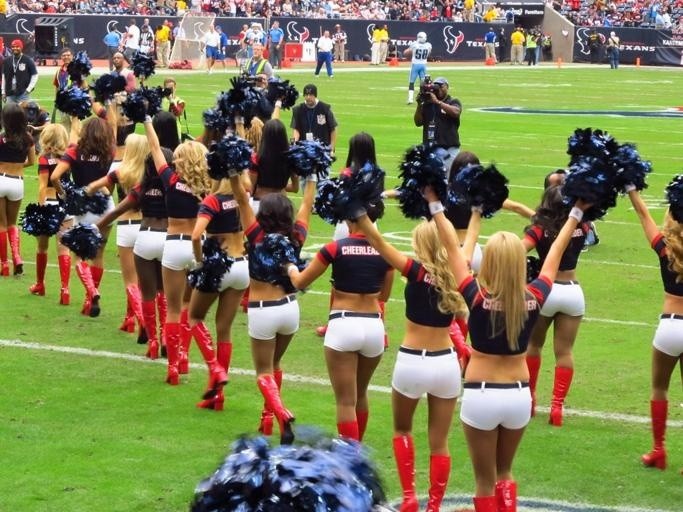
[417,30,427,43]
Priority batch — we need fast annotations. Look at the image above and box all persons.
[1,39,339,446]
[551,1,682,69]
[403,31,433,106]
[315,24,348,77]
[626,178,683,470]
[200,22,228,74]
[415,78,461,185]
[103,18,180,71]
[236,21,284,68]
[1,1,510,22]
[484,24,551,66]
[371,24,390,65]
[286,133,601,512]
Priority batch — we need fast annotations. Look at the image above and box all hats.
[303,84,317,98]
[435,76,449,86]
[11,38,23,47]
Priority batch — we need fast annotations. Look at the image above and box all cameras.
[161,86,171,96]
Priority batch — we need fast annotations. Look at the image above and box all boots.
[119,284,233,411]
[393,434,419,512]
[472,479,519,512]
[257,371,296,444]
[641,400,667,469]
[407,90,415,105]
[0,225,102,318]
[337,412,370,440]
[450,318,469,363]
[525,356,542,416]
[426,456,451,511]
[549,366,573,425]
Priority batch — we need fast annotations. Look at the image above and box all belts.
[399,343,457,358]
[553,277,578,286]
[246,294,296,309]
[233,256,244,261]
[0,171,24,179]
[660,312,683,320]
[462,379,528,389]
[46,196,57,202]
[117,219,204,242]
[328,311,380,321]
